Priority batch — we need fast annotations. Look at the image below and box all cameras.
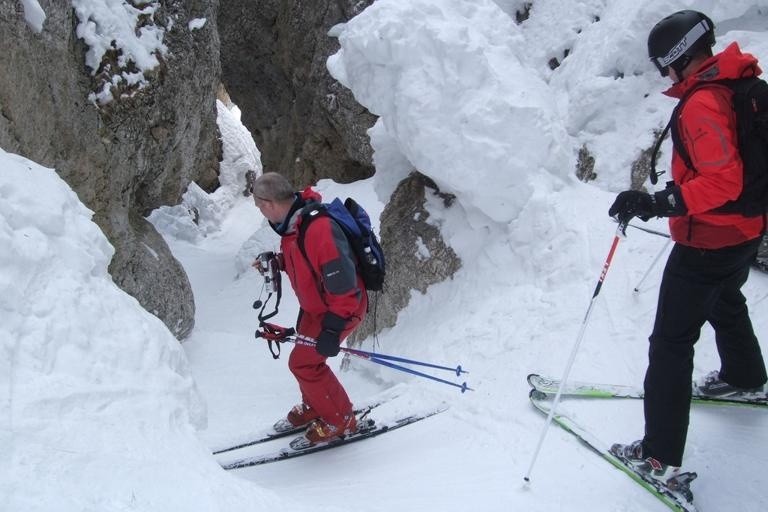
[260,251,277,294]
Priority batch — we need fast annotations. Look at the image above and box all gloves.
[316,311,347,357]
[608,186,684,221]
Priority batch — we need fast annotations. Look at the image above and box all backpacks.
[671,76,767,217]
[297,197,385,291]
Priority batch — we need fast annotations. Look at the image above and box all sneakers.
[618,440,667,476]
[307,413,356,441]
[697,371,768,399]
[287,403,319,425]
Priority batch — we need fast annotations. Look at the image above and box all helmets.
[649,10,716,58]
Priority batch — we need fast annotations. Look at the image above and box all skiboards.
[527,370,767,512]
[212,384,451,470]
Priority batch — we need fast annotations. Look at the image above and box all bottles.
[255,251,280,293]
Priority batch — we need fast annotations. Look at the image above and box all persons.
[249,169,370,448]
[605,9,767,494]
